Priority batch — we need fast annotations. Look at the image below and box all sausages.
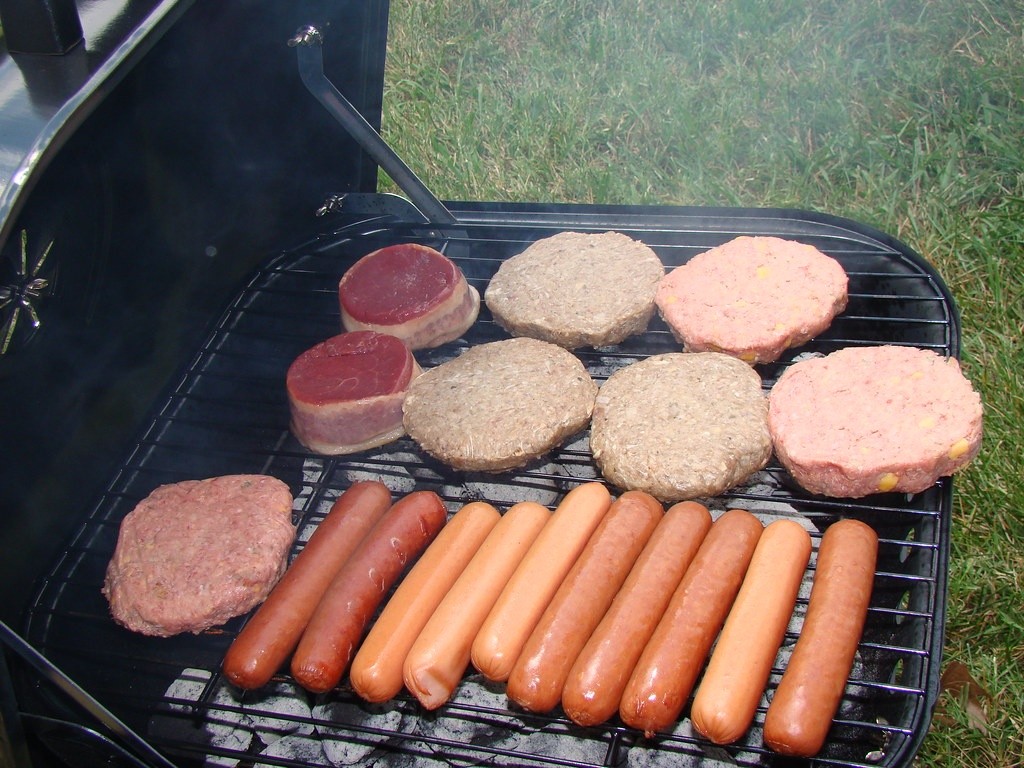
[222,480,878,759]
